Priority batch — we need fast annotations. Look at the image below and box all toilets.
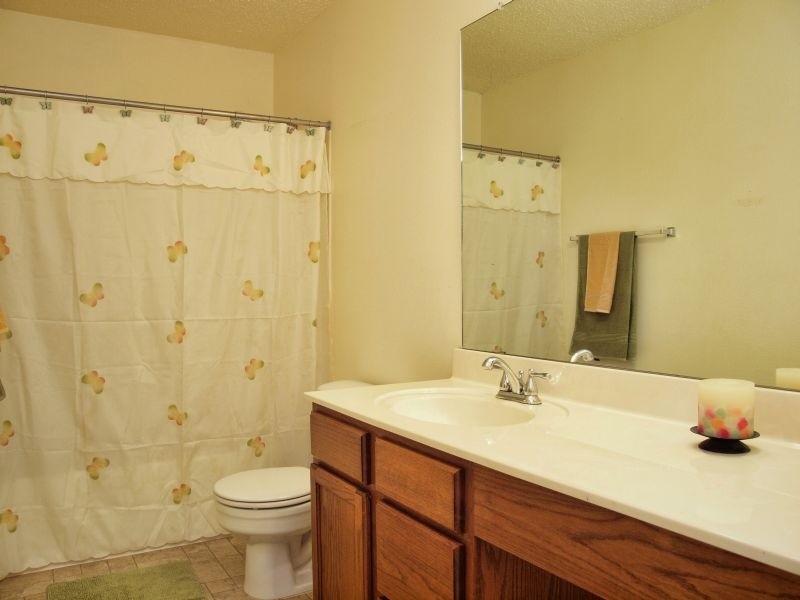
[212,380,376,599]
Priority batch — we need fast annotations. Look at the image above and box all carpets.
[48,560,207,600]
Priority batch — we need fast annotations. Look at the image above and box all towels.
[567,231,639,362]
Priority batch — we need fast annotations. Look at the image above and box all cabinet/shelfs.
[311,402,800,600]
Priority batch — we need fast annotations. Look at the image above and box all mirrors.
[461,0,800,393]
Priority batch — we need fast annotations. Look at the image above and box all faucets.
[481,357,551,405]
[570,349,596,364]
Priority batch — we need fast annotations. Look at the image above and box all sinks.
[379,394,535,428]
